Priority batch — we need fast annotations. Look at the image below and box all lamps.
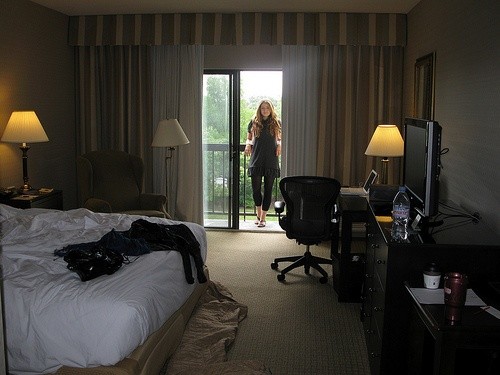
[0,111,50,190]
[152,119,190,219]
[365,125,404,185]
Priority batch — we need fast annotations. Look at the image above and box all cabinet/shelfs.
[359,202,500,375]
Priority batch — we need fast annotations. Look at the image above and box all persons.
[244,99,282,227]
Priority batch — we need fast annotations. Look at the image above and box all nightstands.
[3,189,63,209]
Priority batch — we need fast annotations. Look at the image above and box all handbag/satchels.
[67,244,125,282]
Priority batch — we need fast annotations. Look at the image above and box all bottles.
[391,186,410,239]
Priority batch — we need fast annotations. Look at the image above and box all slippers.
[255,216,266,227]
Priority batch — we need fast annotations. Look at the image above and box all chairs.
[271,176,342,284]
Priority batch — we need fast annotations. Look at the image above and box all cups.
[444,272,468,305]
[417,274,441,289]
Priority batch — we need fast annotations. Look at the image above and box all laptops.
[340,169,378,195]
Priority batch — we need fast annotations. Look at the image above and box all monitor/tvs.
[401,116,443,232]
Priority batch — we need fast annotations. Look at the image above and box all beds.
[0,204,210,375]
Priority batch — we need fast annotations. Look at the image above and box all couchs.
[77,151,167,219]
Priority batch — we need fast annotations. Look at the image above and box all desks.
[330,195,368,259]
